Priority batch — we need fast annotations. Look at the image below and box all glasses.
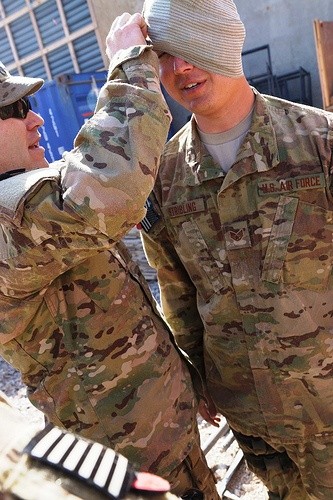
[1,98,33,120]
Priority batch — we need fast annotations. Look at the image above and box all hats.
[0,60,43,108]
[139,0,246,78]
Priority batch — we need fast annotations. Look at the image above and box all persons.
[0,12,223,500]
[136,2,333,500]
[0,395,181,500]
